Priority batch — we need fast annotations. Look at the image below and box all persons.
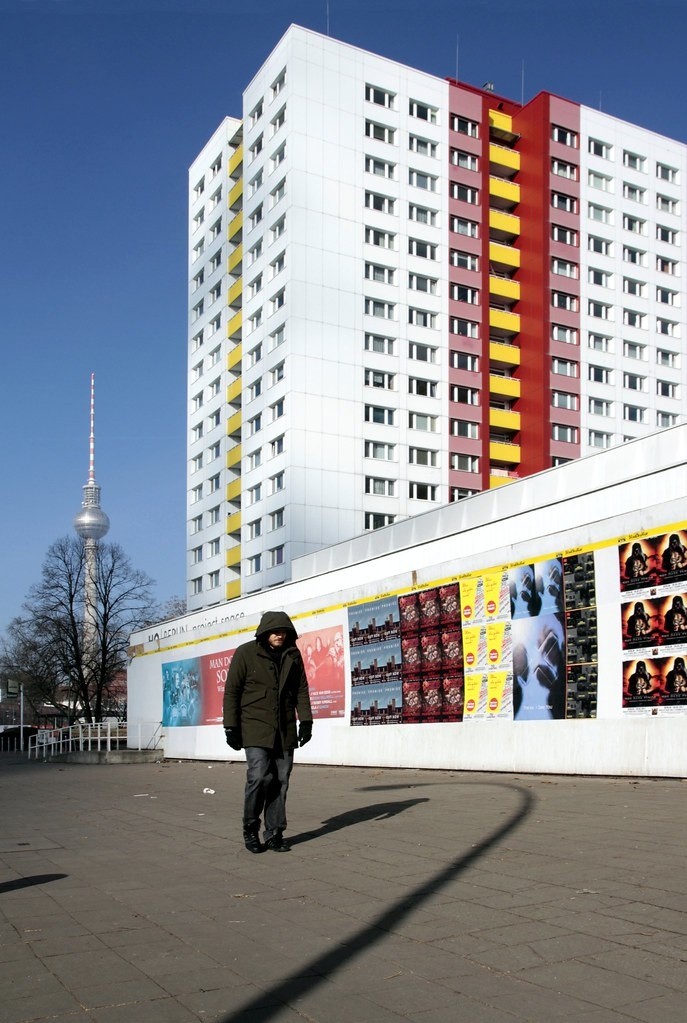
[303,629,343,690]
[623,533,687,706]
[164,667,199,727]
[224,611,314,853]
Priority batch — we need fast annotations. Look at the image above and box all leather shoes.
[244,830,262,852]
[265,839,290,852]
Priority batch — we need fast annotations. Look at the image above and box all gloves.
[298,724,313,746]
[226,731,243,751]
